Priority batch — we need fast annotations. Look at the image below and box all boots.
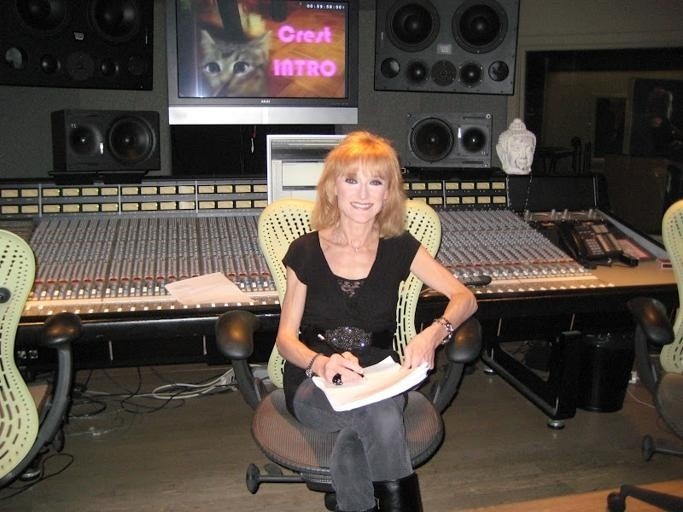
[335,472,424,512]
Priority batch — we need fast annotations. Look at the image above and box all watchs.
[305,353,324,377]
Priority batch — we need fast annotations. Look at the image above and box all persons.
[275,131,479,511]
[495,118,536,175]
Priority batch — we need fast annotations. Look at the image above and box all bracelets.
[432,315,453,345]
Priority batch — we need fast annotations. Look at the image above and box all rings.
[332,373,343,385]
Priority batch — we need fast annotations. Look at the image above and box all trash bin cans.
[574,333,635,413]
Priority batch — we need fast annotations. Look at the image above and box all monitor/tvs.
[265,134,344,208]
[165,1,359,124]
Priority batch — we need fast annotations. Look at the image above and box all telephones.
[558,218,623,261]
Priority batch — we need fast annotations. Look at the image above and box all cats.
[197,12,273,97]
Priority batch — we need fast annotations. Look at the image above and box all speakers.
[374,1,517,97]
[51,109,164,176]
[0,1,154,91]
[401,113,491,170]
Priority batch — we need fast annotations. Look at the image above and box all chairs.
[0,229,82,489]
[215,200,482,512]
[607,198,683,512]
